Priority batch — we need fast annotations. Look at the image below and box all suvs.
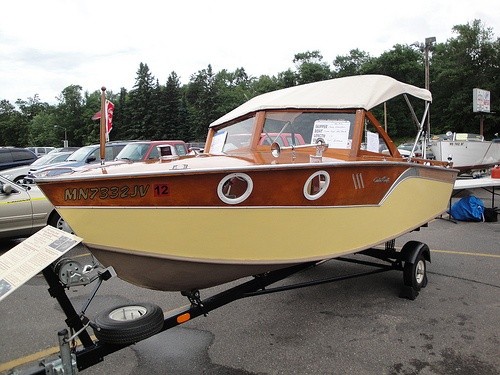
[225,132,305,148]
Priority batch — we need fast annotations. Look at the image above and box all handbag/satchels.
[446,196,486,223]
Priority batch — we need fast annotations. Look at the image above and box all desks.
[435,175,500,224]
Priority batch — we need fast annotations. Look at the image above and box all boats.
[21,73,461,292]
[430,130,500,177]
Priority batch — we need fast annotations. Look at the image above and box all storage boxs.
[491,165,500,179]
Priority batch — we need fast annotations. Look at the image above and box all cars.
[360,136,435,160]
[0,138,205,240]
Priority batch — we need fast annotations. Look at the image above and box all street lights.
[54,125,67,147]
[424,36,437,139]
